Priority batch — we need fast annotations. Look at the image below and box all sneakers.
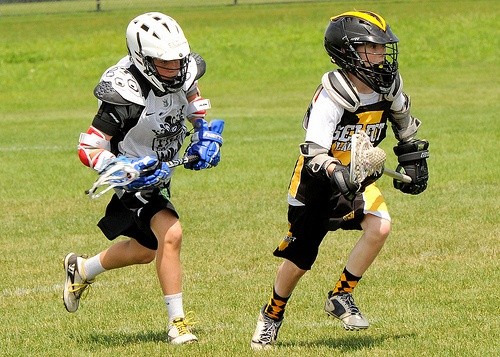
[324,291,370,331]
[250,304,283,350]
[63,252,96,313]
[167,310,199,345]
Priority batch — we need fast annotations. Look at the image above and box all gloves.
[392,140,430,195]
[184,118,225,172]
[103,156,170,192]
[331,163,359,202]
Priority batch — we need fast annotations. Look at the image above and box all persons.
[63,11,225,344]
[252,9,430,349]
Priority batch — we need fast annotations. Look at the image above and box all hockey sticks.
[84,152,200,199]
[349,132,413,192]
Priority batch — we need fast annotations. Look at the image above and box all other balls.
[365,148,386,169]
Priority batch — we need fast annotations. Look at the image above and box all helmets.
[125,12,191,92]
[323,10,400,68]
